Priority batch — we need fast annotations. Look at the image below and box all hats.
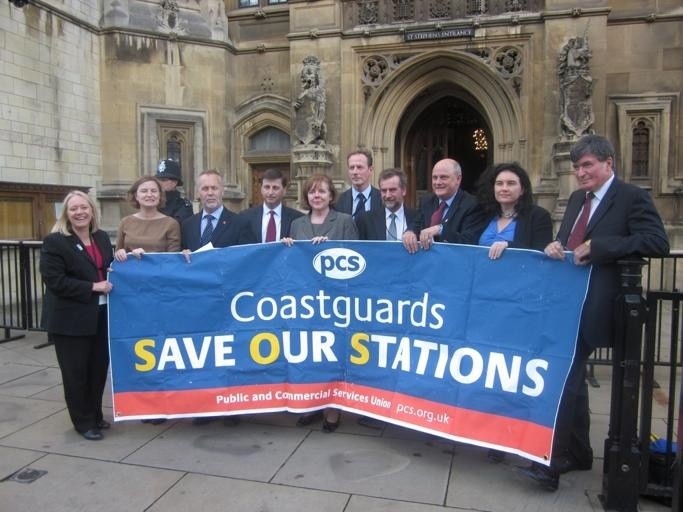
[154,159,184,187]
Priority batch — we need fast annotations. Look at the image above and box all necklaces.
[500,211,516,220]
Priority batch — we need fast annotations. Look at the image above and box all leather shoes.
[323,412,341,432]
[296,410,324,427]
[512,461,560,492]
[560,448,594,474]
[151,419,167,424]
[96,420,110,429]
[82,426,103,440]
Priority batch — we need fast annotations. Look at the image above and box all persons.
[356,168,418,239]
[402,158,481,255]
[238,169,305,243]
[335,150,382,224]
[281,174,359,432]
[475,163,553,464]
[510,135,670,493]
[181,170,259,426]
[155,160,194,224]
[40,190,114,439]
[115,176,181,426]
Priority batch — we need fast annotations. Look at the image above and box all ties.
[354,194,366,222]
[387,213,398,241]
[200,215,214,247]
[430,201,446,227]
[265,210,276,242]
[564,192,596,251]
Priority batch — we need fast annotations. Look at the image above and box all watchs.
[584,239,591,247]
